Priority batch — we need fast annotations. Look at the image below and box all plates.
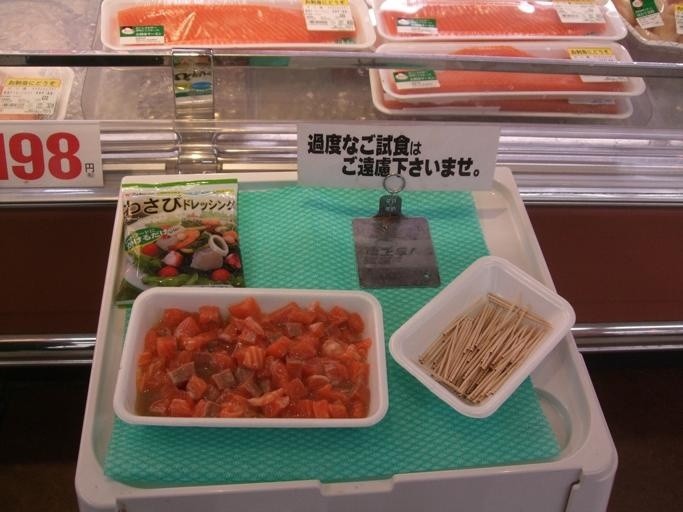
[375,0,631,42]
[113,287,390,429]
[388,254,575,420]
[368,41,647,120]
[612,1,683,49]
[98,0,376,52]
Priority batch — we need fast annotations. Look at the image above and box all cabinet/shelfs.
[1,0,683,373]
